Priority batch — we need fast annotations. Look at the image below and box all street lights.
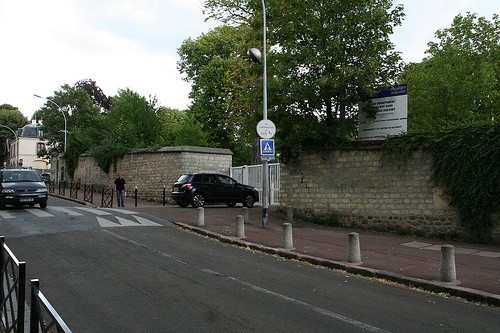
[33,93,67,187]
[248,0,268,229]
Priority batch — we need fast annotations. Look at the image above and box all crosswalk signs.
[260,139,275,156]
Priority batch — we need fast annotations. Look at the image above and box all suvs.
[170,173,259,209]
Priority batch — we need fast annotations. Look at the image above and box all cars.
[40,172,51,184]
[0,166,49,208]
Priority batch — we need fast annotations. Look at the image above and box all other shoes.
[121,206,125,207]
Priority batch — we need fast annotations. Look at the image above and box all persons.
[114,173,127,207]
